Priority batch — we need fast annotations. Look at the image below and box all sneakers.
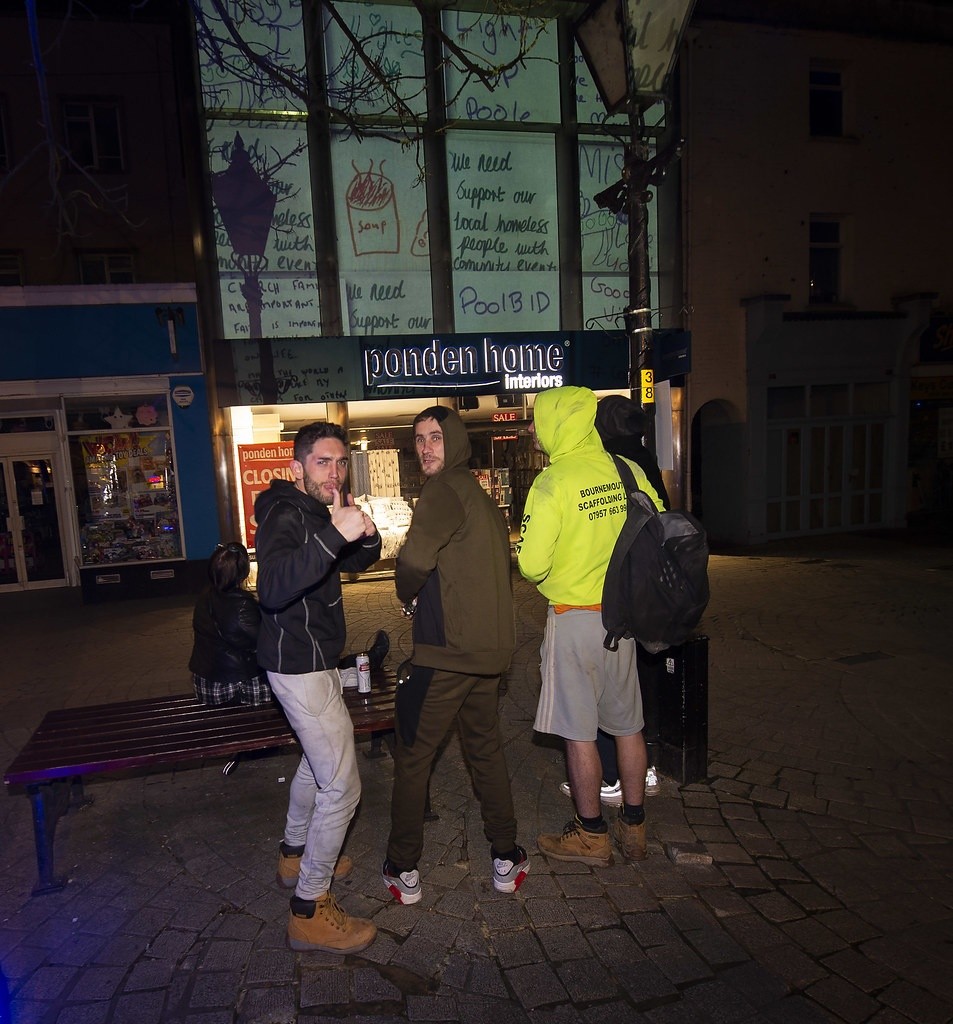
[489,846,530,893]
[613,814,648,861]
[645,765,660,796]
[560,779,623,807]
[286,891,376,955]
[382,859,423,905]
[538,812,612,868]
[277,851,355,887]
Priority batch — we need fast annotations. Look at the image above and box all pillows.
[352,495,416,528]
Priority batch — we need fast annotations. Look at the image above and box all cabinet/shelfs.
[467,436,552,532]
[398,459,432,497]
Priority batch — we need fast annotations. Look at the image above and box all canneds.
[356,653,371,693]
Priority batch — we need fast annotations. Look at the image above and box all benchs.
[2,667,442,899]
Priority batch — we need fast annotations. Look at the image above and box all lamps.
[458,395,479,413]
[494,396,530,410]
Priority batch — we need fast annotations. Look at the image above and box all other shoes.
[369,630,390,670]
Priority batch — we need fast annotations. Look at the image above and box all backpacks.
[601,454,710,654]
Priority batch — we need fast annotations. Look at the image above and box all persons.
[516,385,671,870]
[189,541,391,706]
[382,403,531,906]
[253,421,381,956]
